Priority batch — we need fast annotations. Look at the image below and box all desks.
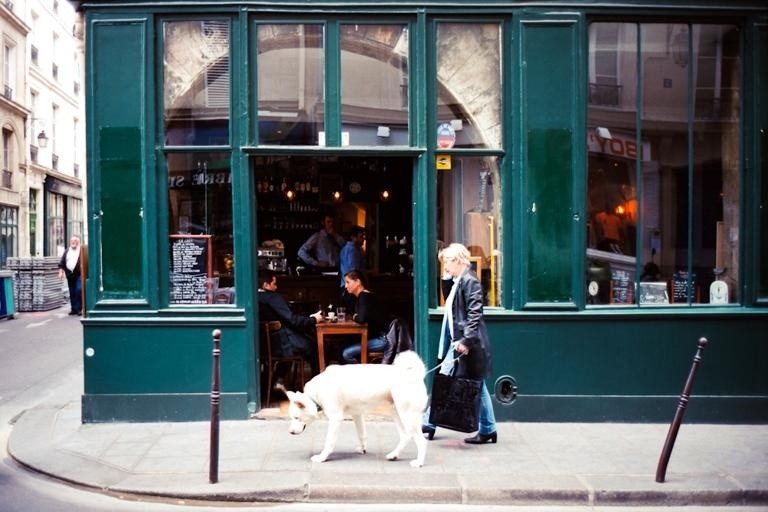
[314,315,369,373]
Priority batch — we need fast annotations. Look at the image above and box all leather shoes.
[69,310,82,315]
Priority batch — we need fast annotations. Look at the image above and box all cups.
[336,307,346,322]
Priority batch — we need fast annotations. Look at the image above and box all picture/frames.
[440,256,481,307]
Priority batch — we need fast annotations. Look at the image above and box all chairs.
[368,312,399,363]
[259,320,304,408]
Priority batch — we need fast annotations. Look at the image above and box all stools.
[287,299,319,317]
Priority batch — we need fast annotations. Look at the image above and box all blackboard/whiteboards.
[611,280,632,303]
[671,269,700,299]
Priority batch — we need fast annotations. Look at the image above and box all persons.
[421,242,498,444]
[594,199,624,254]
[257,214,398,401]
[58,236,83,316]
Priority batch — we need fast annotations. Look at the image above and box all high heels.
[422,425,435,440]
[464,431,497,444]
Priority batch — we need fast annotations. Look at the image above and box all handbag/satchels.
[429,347,483,433]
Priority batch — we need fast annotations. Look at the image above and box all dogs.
[285,348,429,468]
